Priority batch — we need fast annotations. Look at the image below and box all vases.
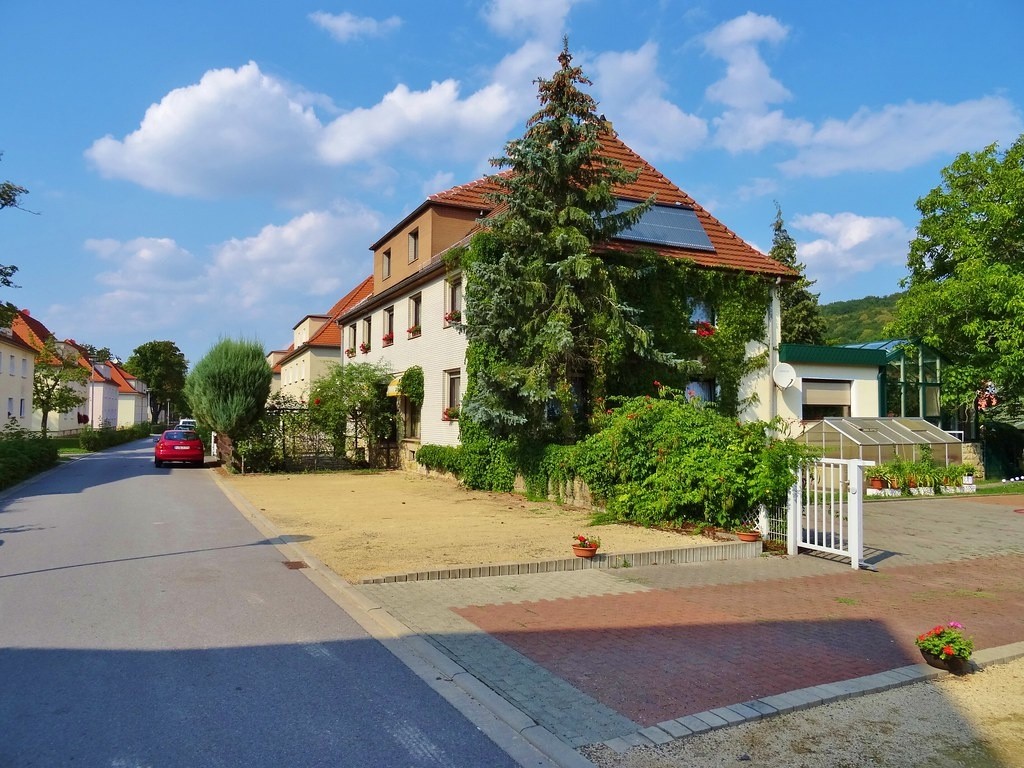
[571,544,597,557]
[412,330,421,336]
[920,648,962,671]
[386,339,393,345]
[362,349,367,353]
[450,316,461,326]
[349,353,356,357]
[736,531,760,542]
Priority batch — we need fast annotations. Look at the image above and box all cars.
[174,425,196,431]
[153,431,205,468]
[179,419,198,425]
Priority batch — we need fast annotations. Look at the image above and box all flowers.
[359,342,370,349]
[345,347,356,354]
[573,533,602,548]
[736,519,755,532]
[444,310,461,322]
[382,330,393,341]
[914,622,975,661]
[444,403,460,419]
[407,324,421,333]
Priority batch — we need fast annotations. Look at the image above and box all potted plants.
[889,463,901,489]
[934,467,950,484]
[907,463,919,488]
[960,461,977,485]
[864,466,891,490]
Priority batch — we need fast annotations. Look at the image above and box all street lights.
[166,398,171,426]
[88,354,99,429]
